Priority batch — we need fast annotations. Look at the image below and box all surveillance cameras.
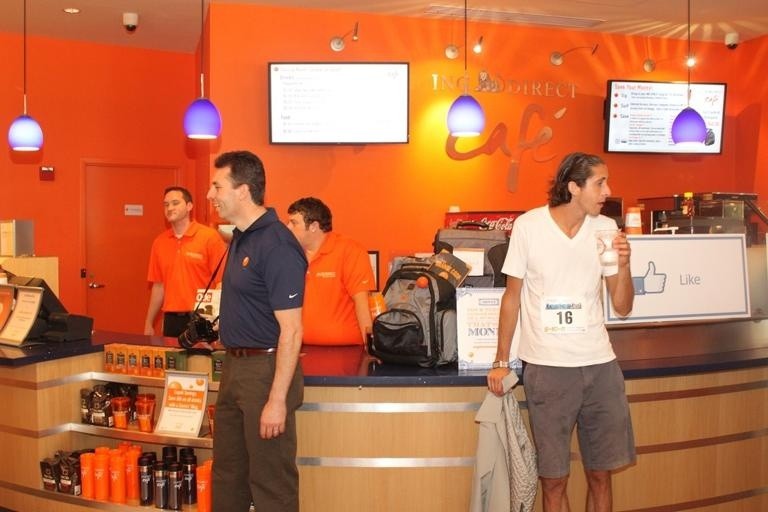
[725,34,739,49]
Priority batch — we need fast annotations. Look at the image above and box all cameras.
[177,318,219,349]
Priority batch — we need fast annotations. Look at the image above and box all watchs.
[492,360,509,369]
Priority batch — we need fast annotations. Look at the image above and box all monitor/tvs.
[267,62,409,145]
[10,277,68,342]
[605,80,727,155]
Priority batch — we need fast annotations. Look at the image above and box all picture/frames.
[364,249,381,293]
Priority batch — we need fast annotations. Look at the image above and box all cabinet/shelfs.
[45,370,222,511]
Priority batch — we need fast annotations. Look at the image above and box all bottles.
[660,211,668,228]
[367,293,386,325]
[80,441,213,511]
[681,192,695,217]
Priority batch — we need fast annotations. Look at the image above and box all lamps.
[6,1,43,154]
[549,42,598,66]
[642,52,697,72]
[328,20,359,53]
[179,0,222,141]
[445,30,484,60]
[444,0,485,138]
[672,0,709,144]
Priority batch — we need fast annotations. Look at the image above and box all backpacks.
[363,255,455,369]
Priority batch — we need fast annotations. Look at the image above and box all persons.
[144,187,228,337]
[286,197,376,348]
[488,152,634,511]
[206,152,308,512]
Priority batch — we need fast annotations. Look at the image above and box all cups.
[592,228,620,276]
[111,396,130,429]
[365,326,377,358]
[207,405,215,438]
[135,393,156,432]
[625,206,643,235]
